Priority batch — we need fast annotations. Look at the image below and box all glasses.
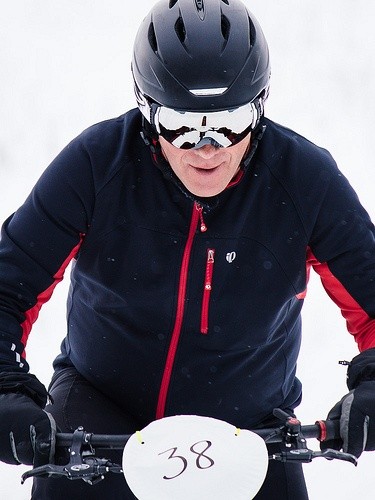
[133,88,268,149]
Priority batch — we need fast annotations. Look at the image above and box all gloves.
[320,350,374,460]
[1,373,72,467]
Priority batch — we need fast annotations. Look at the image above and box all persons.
[0,0,375,500]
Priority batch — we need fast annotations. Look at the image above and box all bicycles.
[1,406,375,488]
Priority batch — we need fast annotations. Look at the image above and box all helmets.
[132,0,271,110]
